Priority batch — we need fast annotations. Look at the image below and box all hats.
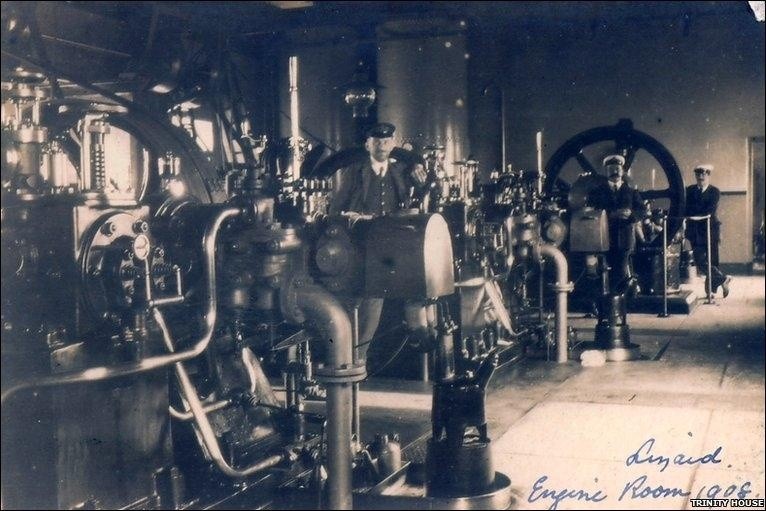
[363,122,397,140]
[692,165,714,175]
[603,154,627,168]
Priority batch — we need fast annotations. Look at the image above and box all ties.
[614,184,618,192]
[376,166,384,179]
[699,187,703,194]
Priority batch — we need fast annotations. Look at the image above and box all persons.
[588,156,647,301]
[682,163,733,301]
[327,122,410,217]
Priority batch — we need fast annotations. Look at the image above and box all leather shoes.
[706,274,732,300]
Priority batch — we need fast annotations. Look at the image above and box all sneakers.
[409,334,423,349]
